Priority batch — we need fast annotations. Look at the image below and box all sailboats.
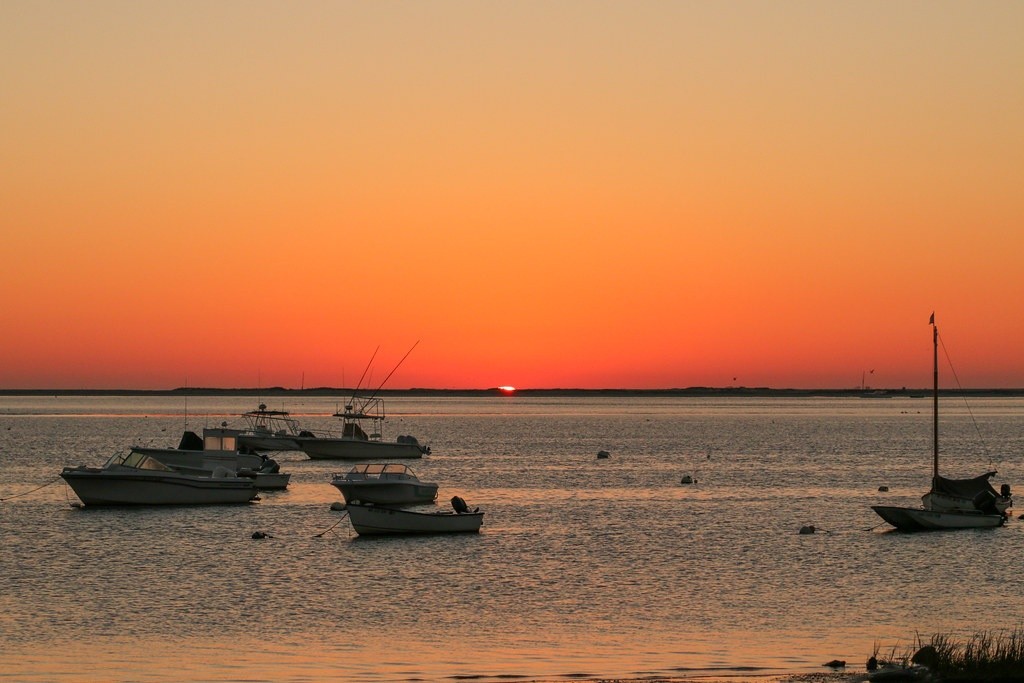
[871,312,1011,532]
[328,340,425,418]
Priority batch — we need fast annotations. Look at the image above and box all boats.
[909,394,924,398]
[246,404,289,416]
[330,464,439,506]
[293,398,431,461]
[254,472,291,491]
[237,434,301,450]
[346,503,485,538]
[864,390,892,398]
[128,428,263,470]
[58,451,258,504]
